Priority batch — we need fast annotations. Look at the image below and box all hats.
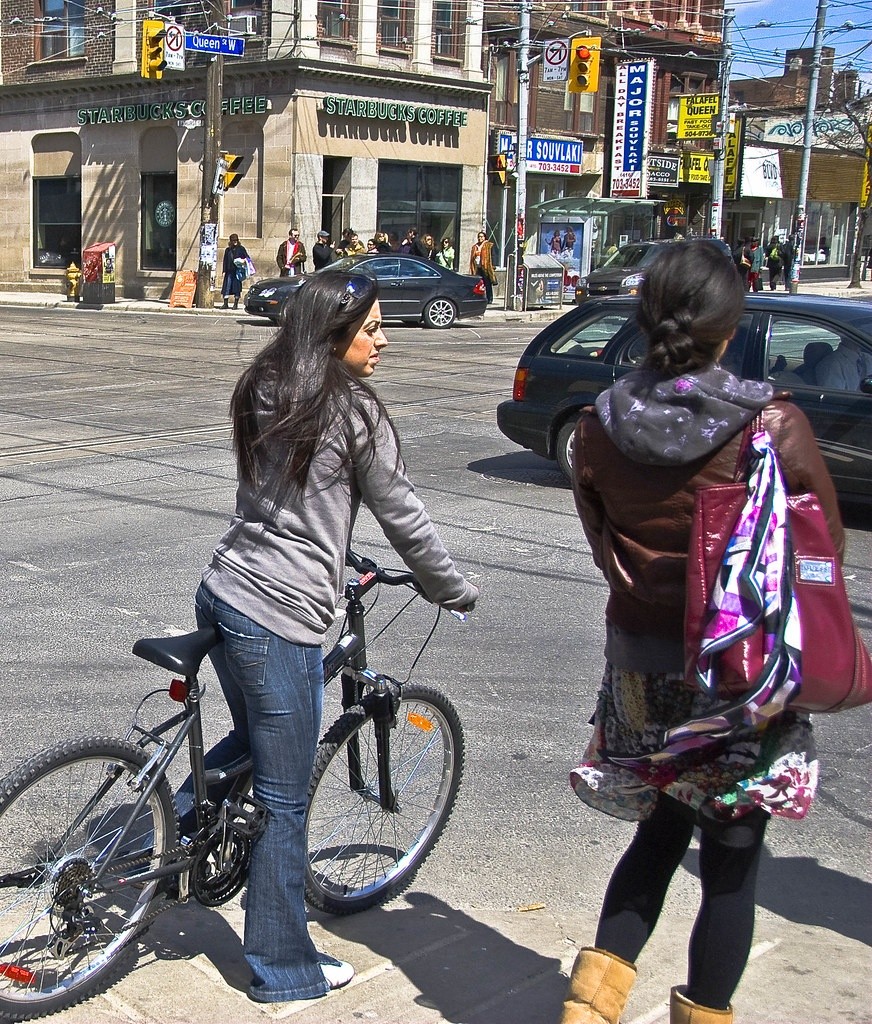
[230,234,239,242]
[317,231,329,237]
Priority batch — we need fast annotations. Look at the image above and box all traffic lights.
[570,36,600,93]
[485,153,507,187]
[143,25,168,77]
[222,154,245,191]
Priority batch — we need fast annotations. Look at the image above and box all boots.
[232,298,241,309]
[671,985,734,1024]
[220,298,228,310]
[557,948,638,1024]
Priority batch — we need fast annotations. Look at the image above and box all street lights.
[786,20,856,292]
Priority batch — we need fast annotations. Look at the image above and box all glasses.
[368,243,373,246]
[426,238,431,241]
[335,272,372,323]
[442,241,448,244]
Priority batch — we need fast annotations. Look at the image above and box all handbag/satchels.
[235,270,245,281]
[683,403,871,712]
[740,247,751,267]
[770,245,780,260]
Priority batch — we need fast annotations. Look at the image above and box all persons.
[600,237,620,266]
[820,236,829,257]
[398,226,419,253]
[731,237,754,290]
[335,228,367,259]
[560,237,845,1024]
[436,238,455,270]
[276,229,307,277]
[373,231,392,253]
[750,241,766,292]
[765,235,785,290]
[124,259,480,1003]
[815,336,872,391]
[470,233,496,303]
[366,239,381,254]
[313,231,335,272]
[720,236,731,253]
[408,233,439,262]
[782,234,795,287]
[545,226,576,259]
[220,234,250,309]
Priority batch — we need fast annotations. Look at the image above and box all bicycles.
[0,544,479,1019]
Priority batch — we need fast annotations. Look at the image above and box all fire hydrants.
[65,261,82,302]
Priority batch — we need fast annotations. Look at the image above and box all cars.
[574,239,673,306]
[243,252,488,330]
[496,289,872,521]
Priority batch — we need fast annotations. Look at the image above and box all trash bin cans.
[83,243,115,304]
[505,253,565,311]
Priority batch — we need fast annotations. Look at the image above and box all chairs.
[768,341,833,387]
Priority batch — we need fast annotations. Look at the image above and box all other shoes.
[319,959,354,991]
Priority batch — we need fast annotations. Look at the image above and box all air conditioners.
[227,14,257,37]
